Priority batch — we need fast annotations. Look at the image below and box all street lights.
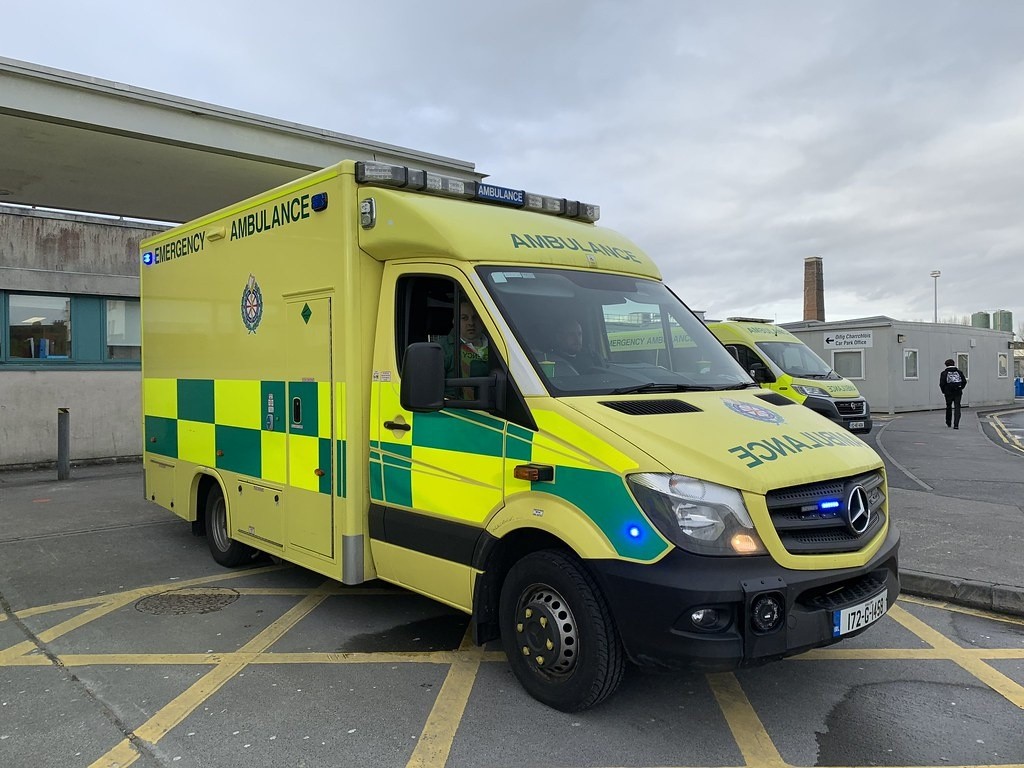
[930,270,941,324]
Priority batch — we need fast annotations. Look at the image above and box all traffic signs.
[824,330,873,349]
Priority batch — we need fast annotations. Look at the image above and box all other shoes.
[946,421,952,426]
[954,425,958,429]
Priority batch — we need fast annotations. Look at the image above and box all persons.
[434,301,491,406]
[939,359,967,429]
[547,318,610,375]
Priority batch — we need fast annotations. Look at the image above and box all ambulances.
[601,315,875,434]
[138,158,901,714]
